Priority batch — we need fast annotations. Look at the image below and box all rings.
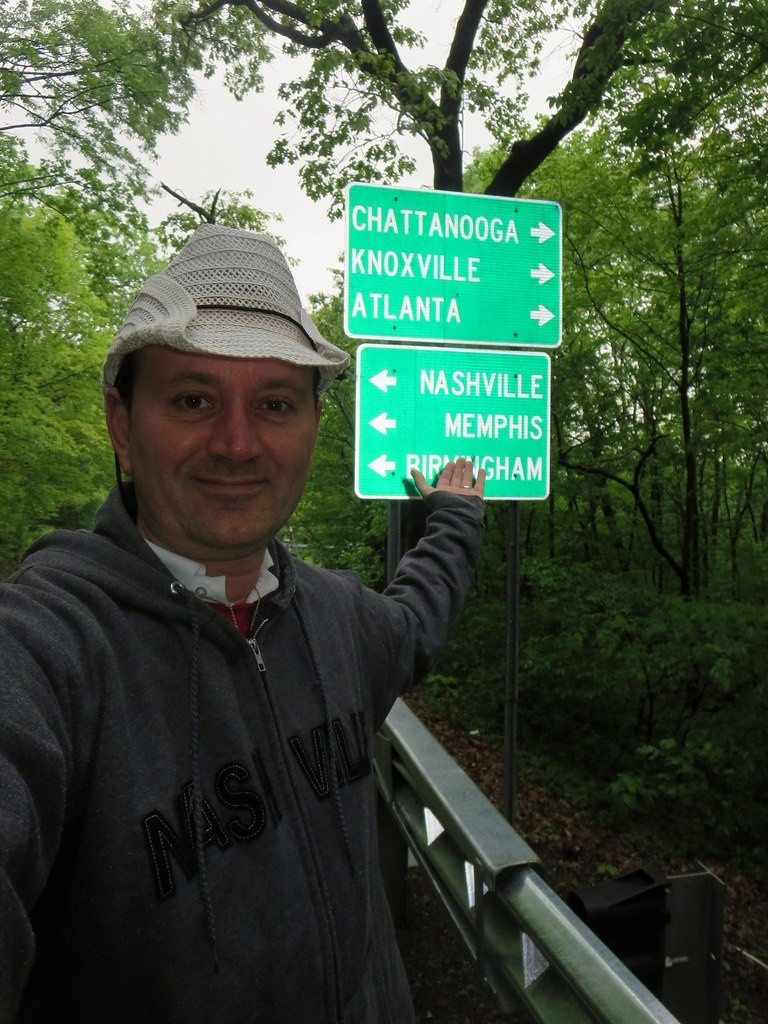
[461,485,473,490]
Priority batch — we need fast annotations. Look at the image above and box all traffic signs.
[354,342,551,501]
[344,183,563,347]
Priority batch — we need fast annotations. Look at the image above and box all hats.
[103,223,351,400]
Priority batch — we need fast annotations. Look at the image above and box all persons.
[1,226,485,1023]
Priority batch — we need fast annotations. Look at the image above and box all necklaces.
[229,585,260,630]
